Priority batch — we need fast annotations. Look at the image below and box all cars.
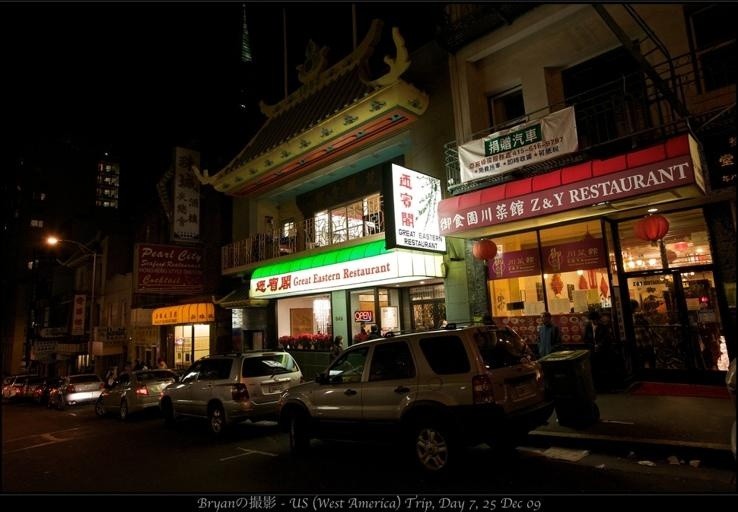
[0,324,555,473]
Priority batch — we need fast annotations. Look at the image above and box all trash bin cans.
[538,350,600,429]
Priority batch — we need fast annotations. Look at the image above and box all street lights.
[47,237,96,368]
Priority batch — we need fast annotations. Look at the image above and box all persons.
[583,311,613,394]
[537,310,564,358]
[104,356,168,387]
[481,311,496,324]
[629,298,639,314]
[327,334,346,367]
[368,325,380,340]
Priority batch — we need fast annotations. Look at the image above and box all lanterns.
[472,237,498,267]
[634,211,671,248]
